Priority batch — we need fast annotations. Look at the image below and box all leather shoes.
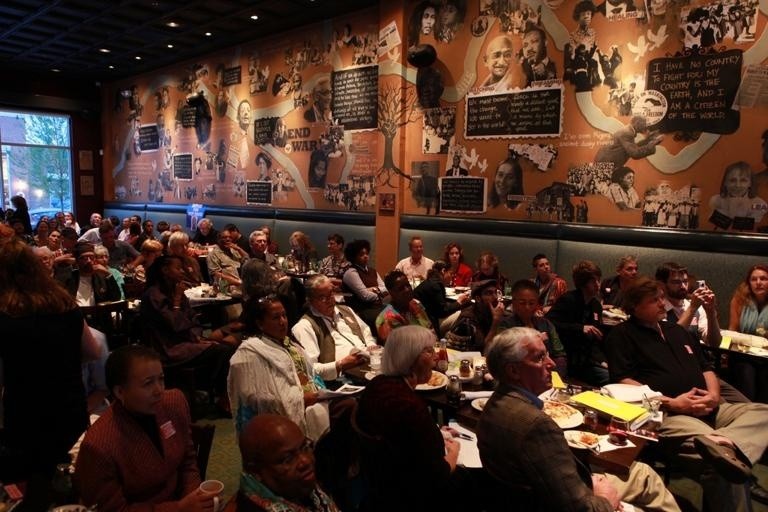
[745,480,768,505]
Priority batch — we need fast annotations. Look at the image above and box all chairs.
[0,206,767,512]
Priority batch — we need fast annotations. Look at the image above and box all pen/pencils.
[439,424,473,439]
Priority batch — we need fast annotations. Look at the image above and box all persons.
[0,197,767,511]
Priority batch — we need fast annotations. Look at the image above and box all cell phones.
[696,279,706,290]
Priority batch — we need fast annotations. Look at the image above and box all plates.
[412,369,450,391]
[365,370,378,381]
[470,396,584,429]
[560,429,599,450]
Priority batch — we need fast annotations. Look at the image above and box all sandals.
[693,435,751,485]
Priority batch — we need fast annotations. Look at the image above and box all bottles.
[445,360,482,398]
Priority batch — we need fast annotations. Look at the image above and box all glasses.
[79,254,95,261]
[265,436,314,472]
[519,350,550,363]
[314,290,338,302]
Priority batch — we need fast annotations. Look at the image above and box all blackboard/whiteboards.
[438,176,488,214]
[138,123,161,153]
[246,180,273,206]
[333,65,379,133]
[222,65,241,86]
[172,153,193,181]
[254,116,280,145]
[462,83,565,140]
[647,49,744,135]
[182,107,196,127]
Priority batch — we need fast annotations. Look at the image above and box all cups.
[200,480,225,512]
[584,408,598,429]
[609,414,629,446]
